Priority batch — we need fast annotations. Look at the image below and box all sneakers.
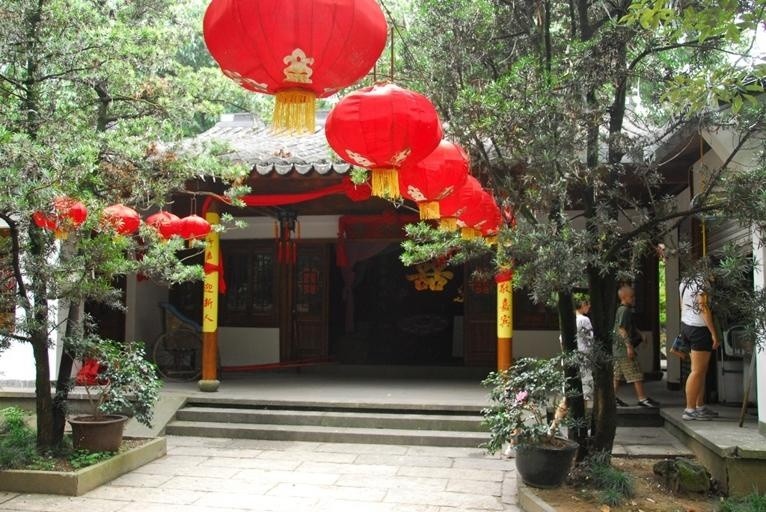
[638,398,661,409]
[547,428,568,440]
[681,406,719,421]
[614,397,628,407]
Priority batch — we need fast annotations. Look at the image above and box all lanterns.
[471,205,498,231]
[324,84,443,199]
[459,193,492,240]
[33,202,211,251]
[203,0,388,133]
[391,140,467,220]
[416,174,482,232]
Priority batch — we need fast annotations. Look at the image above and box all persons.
[613,286,660,409]
[679,255,719,421]
[548,292,594,435]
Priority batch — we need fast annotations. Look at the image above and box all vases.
[68,415,128,455]
[515,436,580,488]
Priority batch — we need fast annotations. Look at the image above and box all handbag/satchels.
[670,335,691,360]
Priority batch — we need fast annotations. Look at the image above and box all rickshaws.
[152,302,338,383]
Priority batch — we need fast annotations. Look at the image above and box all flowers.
[479,351,589,454]
[60,336,164,429]
